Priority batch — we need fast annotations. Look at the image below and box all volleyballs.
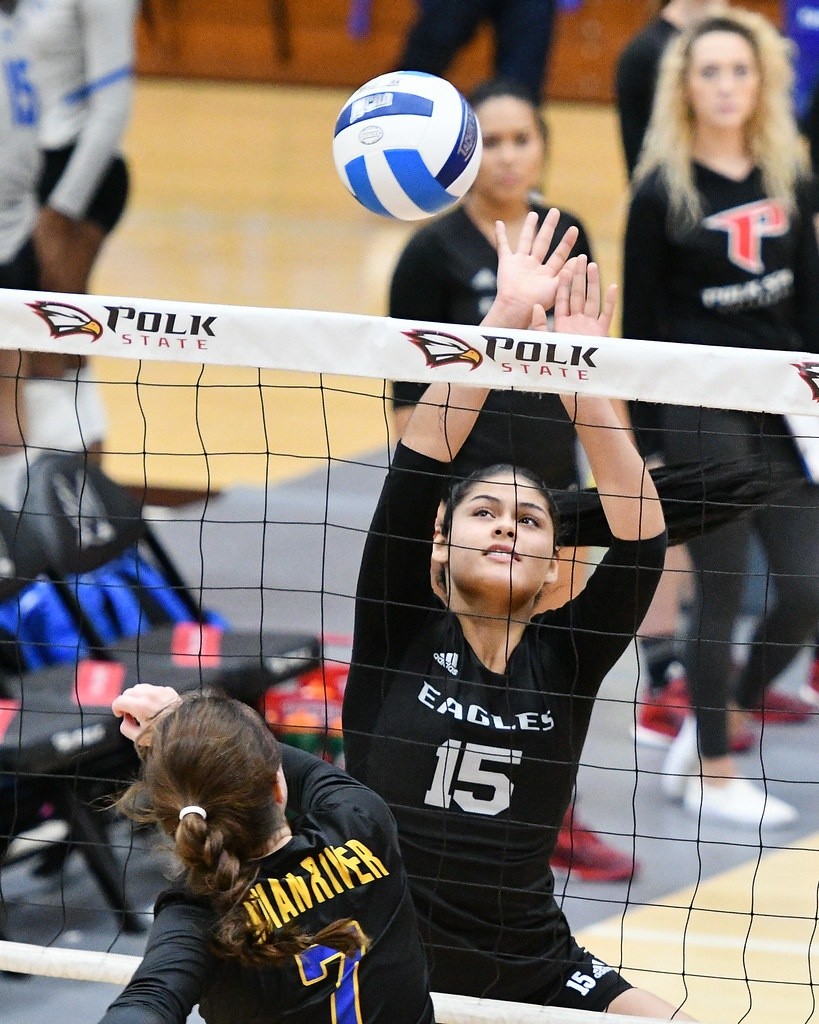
[330,69,486,226]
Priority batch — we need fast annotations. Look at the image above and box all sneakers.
[632,693,755,752]
[660,713,694,800]
[797,659,819,709]
[682,773,799,829]
[548,804,641,881]
[759,683,819,722]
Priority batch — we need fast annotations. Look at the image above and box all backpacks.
[6,550,241,671]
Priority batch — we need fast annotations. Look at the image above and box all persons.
[1,0,137,473]
[388,76,640,885]
[100,683,436,1024]
[611,0,819,823]
[341,206,703,1023]
[390,0,557,103]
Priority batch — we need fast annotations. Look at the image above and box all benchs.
[0,446,321,934]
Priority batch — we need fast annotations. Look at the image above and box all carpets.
[0,448,819,1024]
